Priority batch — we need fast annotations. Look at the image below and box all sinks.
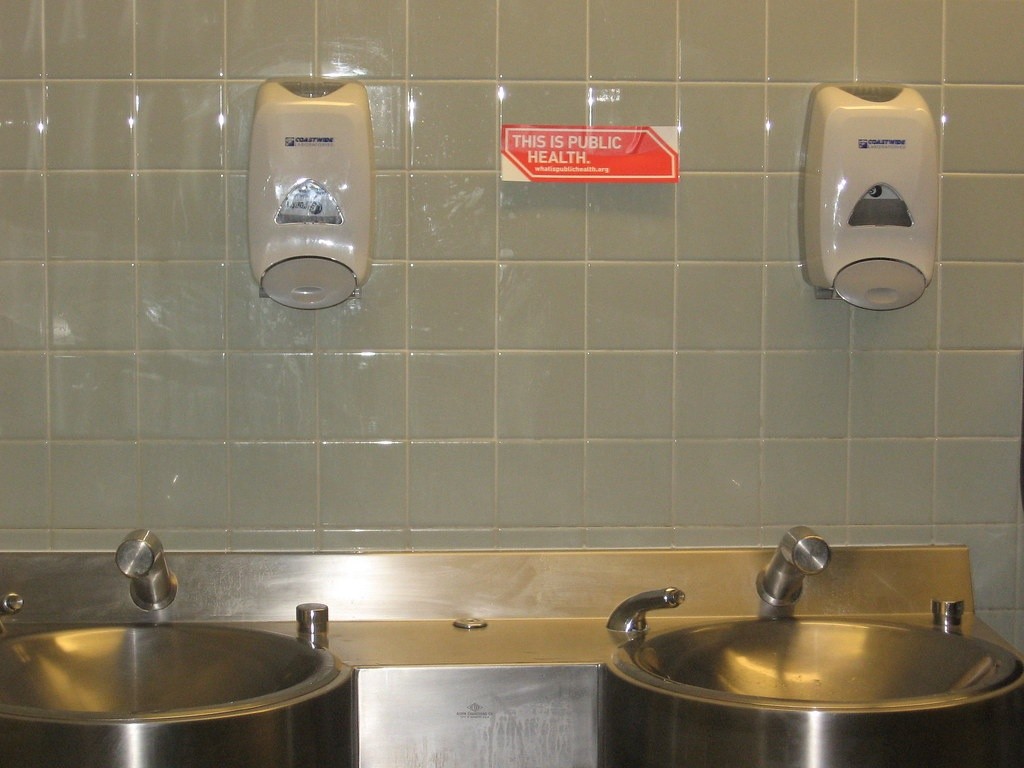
[608,613,1023,768]
[0,621,355,768]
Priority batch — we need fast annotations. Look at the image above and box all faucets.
[112,527,180,611]
[756,525,833,608]
